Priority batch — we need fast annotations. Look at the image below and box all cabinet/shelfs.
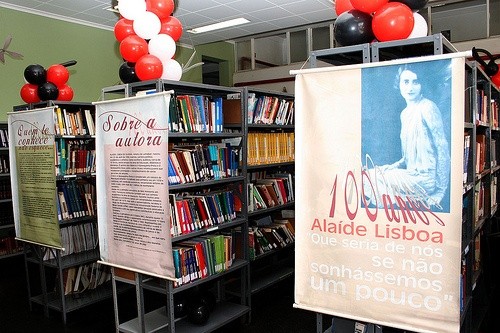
[0,122,31,259]
[102,79,296,333]
[371,33,500,333]
[14,100,131,333]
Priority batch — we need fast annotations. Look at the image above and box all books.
[0,91,295,294]
[460,80,500,313]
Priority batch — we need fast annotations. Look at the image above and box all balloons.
[114,0,182,84]
[333,0,430,46]
[20,64,73,102]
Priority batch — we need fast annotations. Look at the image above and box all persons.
[362,63,450,206]
